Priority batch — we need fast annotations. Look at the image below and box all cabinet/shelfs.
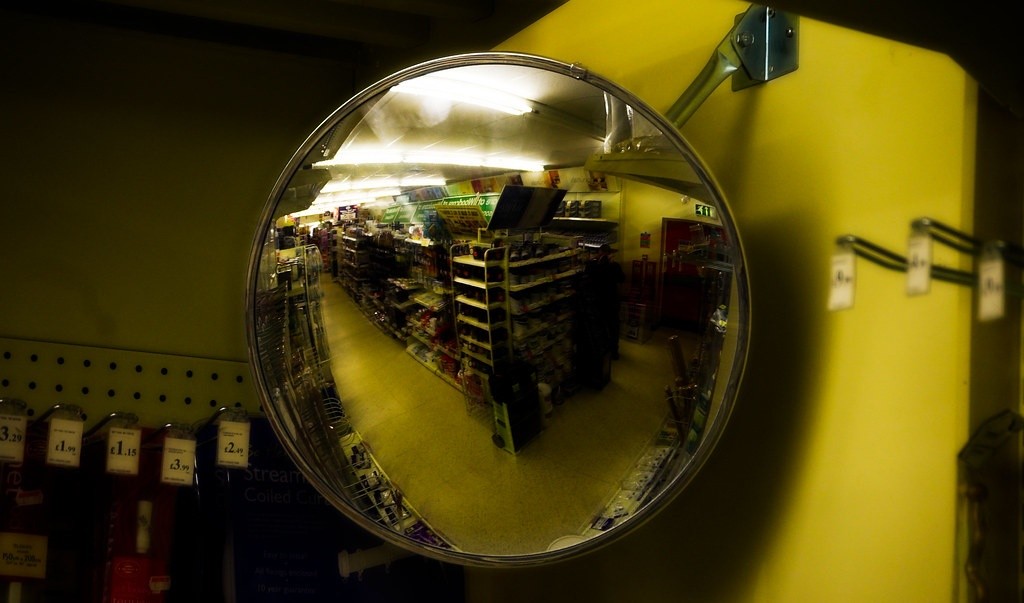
[326,223,592,416]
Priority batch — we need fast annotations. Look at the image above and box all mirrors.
[242,52,754,572]
